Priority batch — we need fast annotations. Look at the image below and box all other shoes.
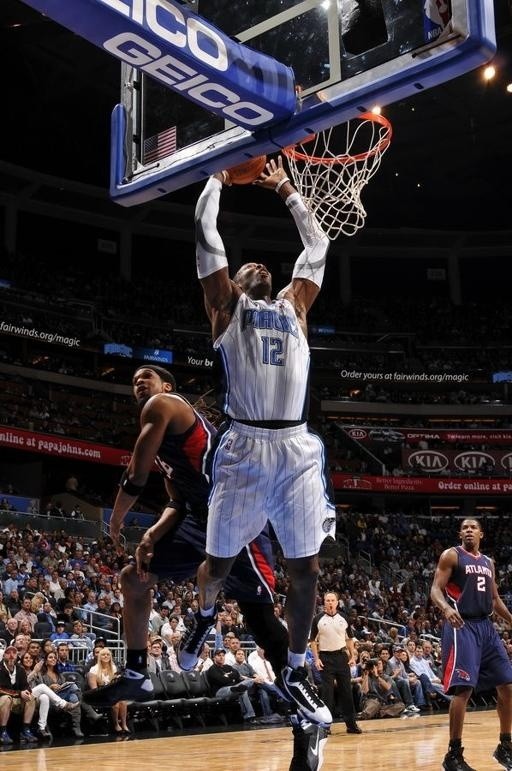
[419,705,433,711]
[408,705,421,712]
[347,725,362,733]
[401,709,409,714]
[230,684,246,693]
[244,718,260,726]
[113,727,132,736]
[426,690,437,697]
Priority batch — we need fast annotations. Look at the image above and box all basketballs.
[225,153,266,185]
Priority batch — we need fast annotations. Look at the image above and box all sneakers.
[442,746,474,770]
[82,663,154,707]
[19,730,37,742]
[274,664,335,728]
[494,742,511,769]
[0,731,13,743]
[286,707,328,771]
[175,607,218,671]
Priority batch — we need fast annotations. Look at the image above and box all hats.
[57,621,65,629]
[215,648,226,655]
[94,637,106,645]
[6,646,17,653]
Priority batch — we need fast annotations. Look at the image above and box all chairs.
[0,525,512,740]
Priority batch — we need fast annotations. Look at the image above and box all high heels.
[36,727,50,738]
[63,701,80,715]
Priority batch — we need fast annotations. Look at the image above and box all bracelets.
[219,169,229,184]
[272,175,292,193]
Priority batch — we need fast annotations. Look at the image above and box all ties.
[264,661,271,681]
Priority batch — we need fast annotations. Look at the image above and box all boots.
[77,688,103,723]
[71,700,84,738]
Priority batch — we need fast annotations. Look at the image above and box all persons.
[87,360,331,771]
[2,276,512,749]
[175,154,345,730]
[430,516,512,771]
